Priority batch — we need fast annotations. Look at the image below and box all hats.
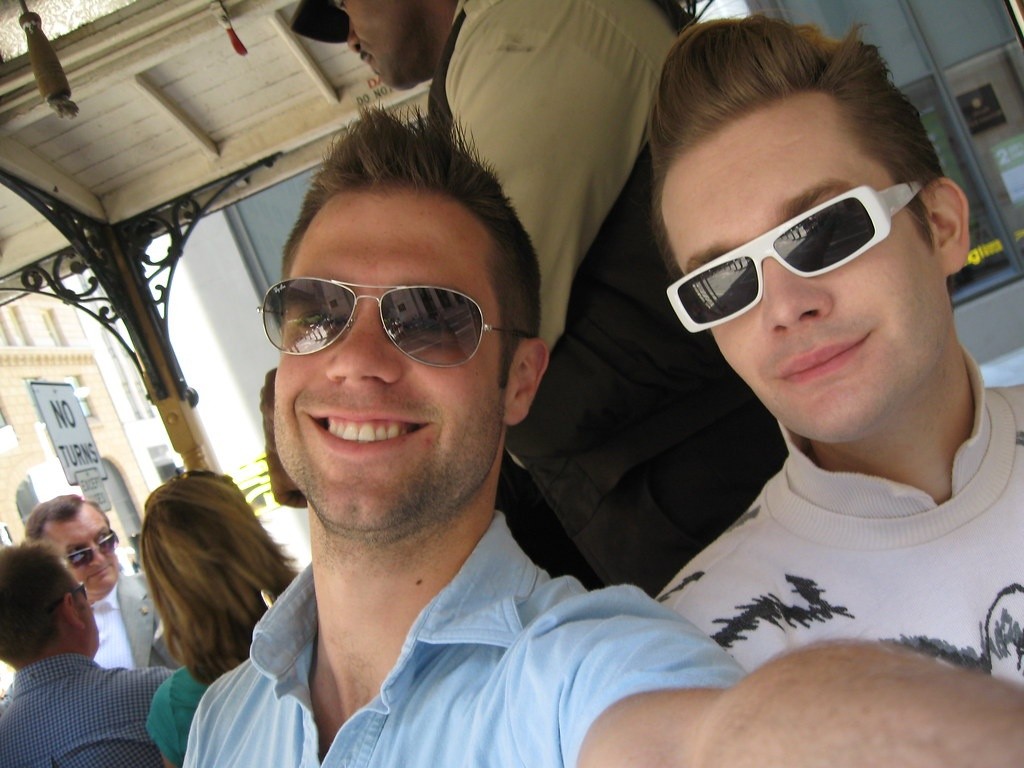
[288,1,349,43]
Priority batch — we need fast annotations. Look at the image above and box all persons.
[0,541,177,768]
[24,493,178,671]
[647,15,1024,678]
[262,0,785,601]
[179,103,1024,768]
[142,470,303,768]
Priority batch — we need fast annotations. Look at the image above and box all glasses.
[257,276,533,368]
[169,469,216,484]
[666,181,922,335]
[67,529,120,568]
[48,583,87,612]
